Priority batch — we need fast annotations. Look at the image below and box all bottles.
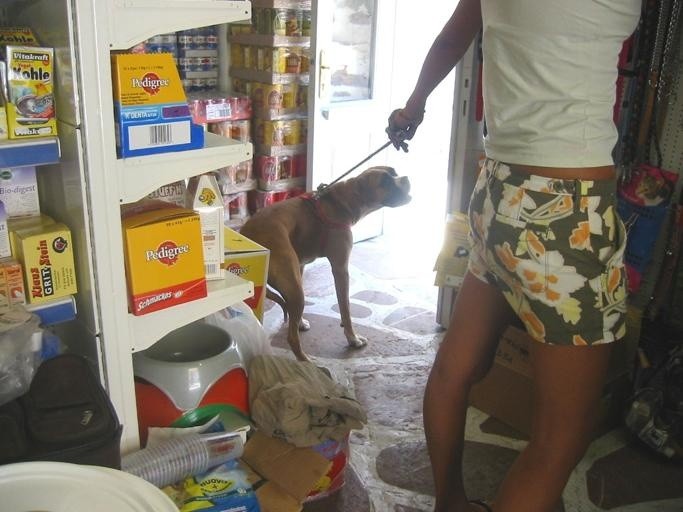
[254,154,306,180]
[234,80,308,108]
[147,33,177,57]
[230,24,256,37]
[249,188,306,215]
[222,193,246,221]
[177,57,219,78]
[201,120,251,145]
[183,90,252,124]
[177,27,218,56]
[252,7,313,36]
[253,118,308,146]
[220,160,252,185]
[183,80,219,93]
[231,43,311,75]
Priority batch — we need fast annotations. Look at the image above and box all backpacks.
[27,356,120,468]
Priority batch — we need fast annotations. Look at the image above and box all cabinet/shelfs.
[137,0,314,235]
[1,0,253,464]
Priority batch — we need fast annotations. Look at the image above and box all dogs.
[239,166,411,364]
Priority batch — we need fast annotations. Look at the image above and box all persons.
[386,0,642,512]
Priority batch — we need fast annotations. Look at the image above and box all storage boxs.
[220,224,269,326]
[1,353,123,471]
[120,197,207,317]
